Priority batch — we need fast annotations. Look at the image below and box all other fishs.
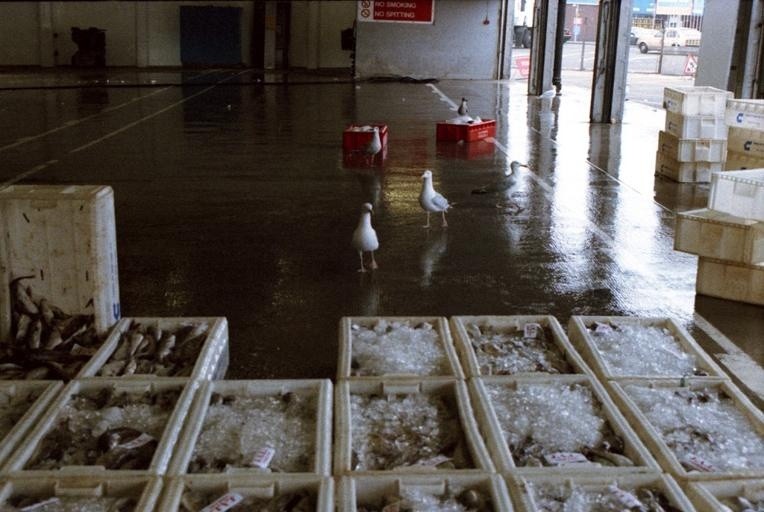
[28,383,181,470]
[0,300,209,380]
[11,275,39,315]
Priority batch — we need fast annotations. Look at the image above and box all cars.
[637,26,701,55]
[630,27,658,46]
[563,25,573,48]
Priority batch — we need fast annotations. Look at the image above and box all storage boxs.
[434,116,497,147]
[674,172,756,306]
[0,315,763,512]
[0,184,121,380]
[652,85,763,184]
[342,122,387,157]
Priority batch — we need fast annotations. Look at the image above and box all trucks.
[513,0,534,50]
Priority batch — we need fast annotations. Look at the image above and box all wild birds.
[458,97,470,115]
[468,161,527,210]
[353,202,379,274]
[539,85,557,101]
[359,126,382,168]
[419,169,458,228]
